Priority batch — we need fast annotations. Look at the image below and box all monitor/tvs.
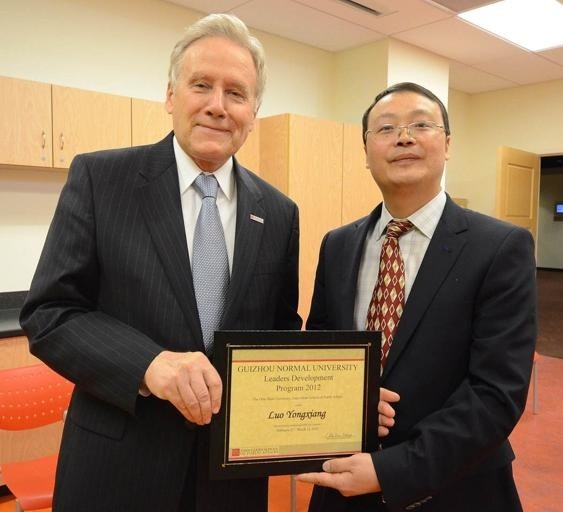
[554,203,563,215]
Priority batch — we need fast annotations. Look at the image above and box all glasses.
[364,119,446,140]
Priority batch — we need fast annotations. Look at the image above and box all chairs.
[0,363,78,512]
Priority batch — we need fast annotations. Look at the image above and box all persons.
[20,13,401,512]
[294,83,537,512]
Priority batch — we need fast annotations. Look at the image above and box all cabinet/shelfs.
[0,76,175,176]
[262,112,384,329]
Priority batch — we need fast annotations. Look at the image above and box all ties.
[189,172,231,358]
[363,219,415,378]
